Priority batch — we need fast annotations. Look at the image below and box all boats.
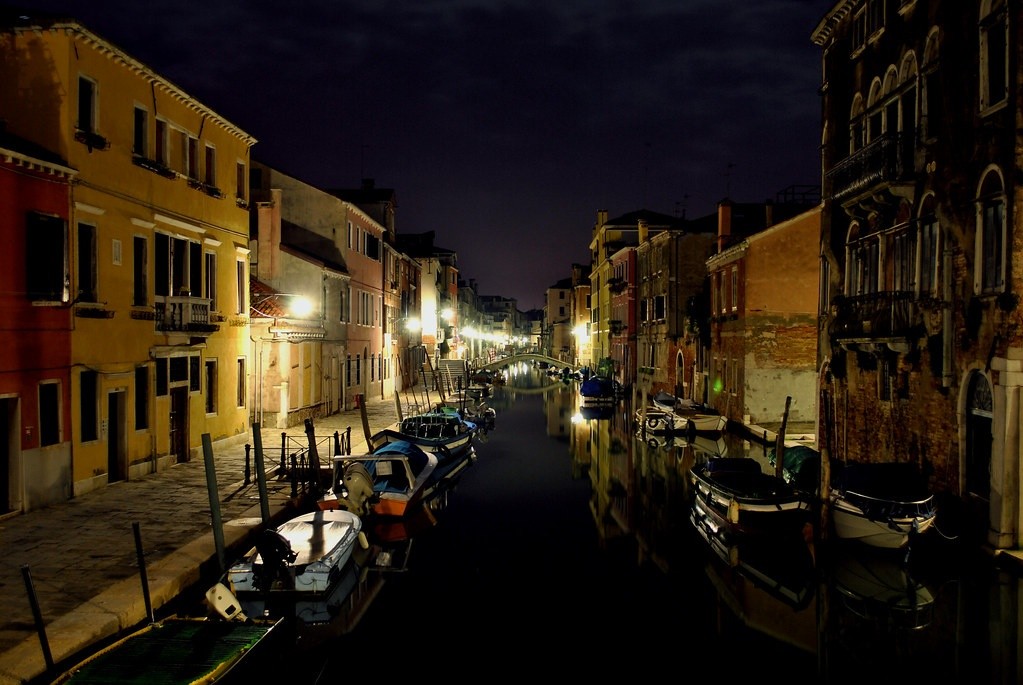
[580,378,614,401]
[688,454,811,521]
[466,369,505,395]
[636,405,671,432]
[688,495,818,610]
[316,438,439,523]
[370,352,478,465]
[436,361,495,427]
[548,363,597,380]
[768,444,938,551]
[38,585,327,685]
[216,506,362,593]
[822,522,948,632]
[652,389,729,432]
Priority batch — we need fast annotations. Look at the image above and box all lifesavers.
[437,443,452,457]
[647,438,658,449]
[647,417,660,428]
